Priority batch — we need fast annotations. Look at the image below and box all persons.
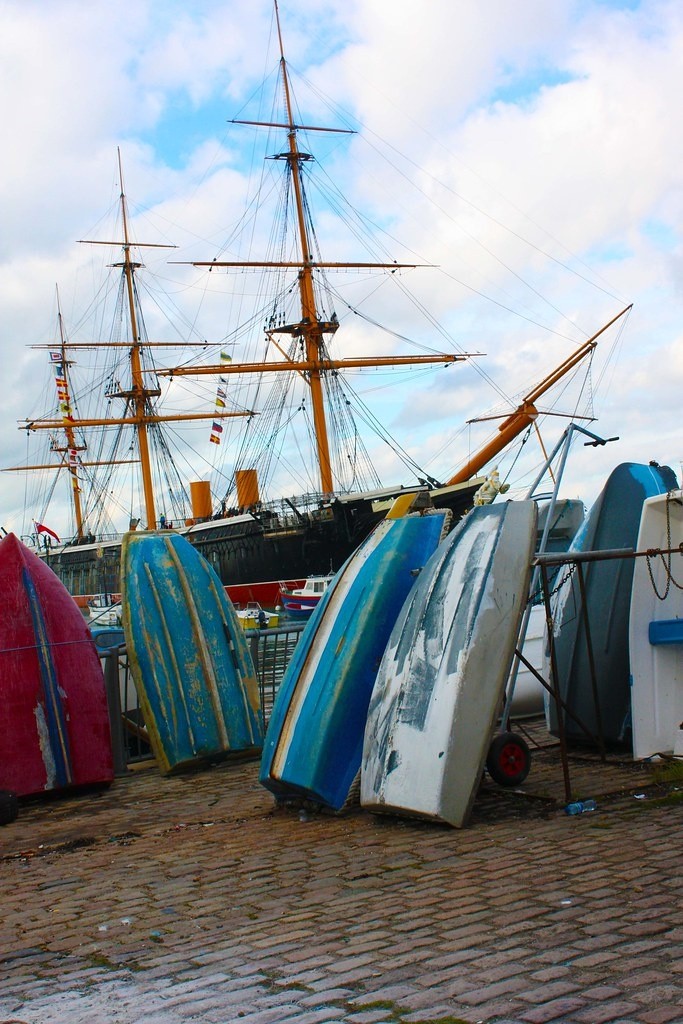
[159,512,166,529]
[86,597,92,607]
[47,536,52,549]
[43,535,47,549]
[87,528,93,544]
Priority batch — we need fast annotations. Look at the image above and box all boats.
[542,459,670,752]
[259,502,456,812]
[1,529,116,812]
[278,558,338,619]
[87,593,122,627]
[120,528,266,779]
[235,602,279,630]
[360,494,537,827]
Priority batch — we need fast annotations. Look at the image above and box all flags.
[215,410,224,420]
[72,482,82,493]
[210,434,220,444]
[70,460,79,467]
[212,422,222,433]
[67,448,79,456]
[63,416,75,423]
[58,391,70,400]
[49,352,62,360]
[70,470,82,480]
[216,398,225,407]
[220,351,232,362]
[217,387,226,398]
[34,521,61,544]
[60,402,73,413]
[56,378,68,387]
[65,428,75,439]
[56,366,64,376]
[219,376,228,385]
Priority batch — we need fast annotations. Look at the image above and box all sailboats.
[22,56,618,634]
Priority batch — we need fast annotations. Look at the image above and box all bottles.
[563,799,599,815]
